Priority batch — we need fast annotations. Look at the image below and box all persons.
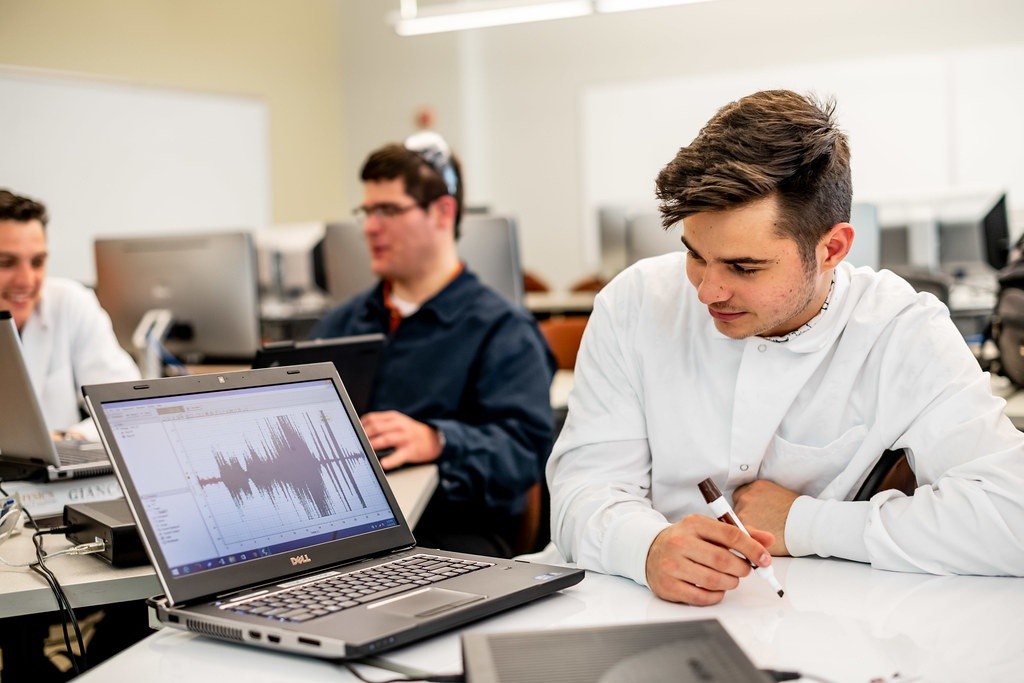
[303,140,560,562]
[0,188,161,683]
[545,87,1024,607]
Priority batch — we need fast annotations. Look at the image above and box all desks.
[526,293,599,318]
[67,556,1023,682]
[260,293,335,340]
[1,464,438,626]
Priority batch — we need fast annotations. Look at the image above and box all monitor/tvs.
[95,231,264,362]
[322,214,525,306]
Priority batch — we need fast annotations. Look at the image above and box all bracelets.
[55,429,75,441]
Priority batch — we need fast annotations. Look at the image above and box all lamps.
[391,1,697,35]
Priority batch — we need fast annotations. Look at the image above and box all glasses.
[354,204,416,223]
[405,130,458,196]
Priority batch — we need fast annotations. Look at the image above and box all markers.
[696,477,761,568]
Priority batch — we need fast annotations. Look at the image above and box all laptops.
[250,332,390,466]
[598,191,1023,274]
[0,309,114,483]
[79,359,586,662]
